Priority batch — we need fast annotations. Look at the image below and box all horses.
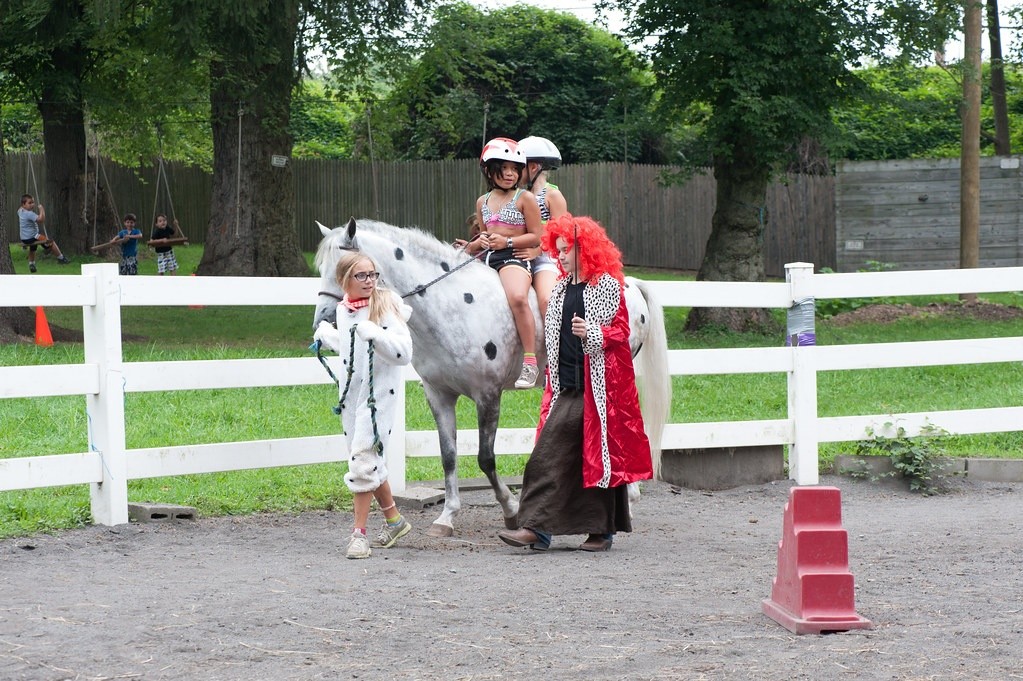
[313,216,672,540]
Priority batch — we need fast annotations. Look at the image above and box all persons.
[17,194,70,273]
[150,214,178,275]
[110,213,142,274]
[313,251,413,559]
[497,213,654,552]
[456,135,567,389]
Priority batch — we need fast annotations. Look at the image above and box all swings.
[146,124,189,246]
[91,125,130,255]
[17,126,53,250]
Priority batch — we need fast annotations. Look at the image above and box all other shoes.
[57,256,72,264]
[29,264,37,273]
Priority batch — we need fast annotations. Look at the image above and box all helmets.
[479,137,527,169]
[517,135,562,170]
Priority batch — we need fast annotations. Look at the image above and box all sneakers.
[369,514,411,548]
[346,529,371,559]
[515,363,539,388]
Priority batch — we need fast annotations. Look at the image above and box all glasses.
[348,271,379,281]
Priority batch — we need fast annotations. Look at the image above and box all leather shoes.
[498,527,549,550]
[579,535,613,551]
